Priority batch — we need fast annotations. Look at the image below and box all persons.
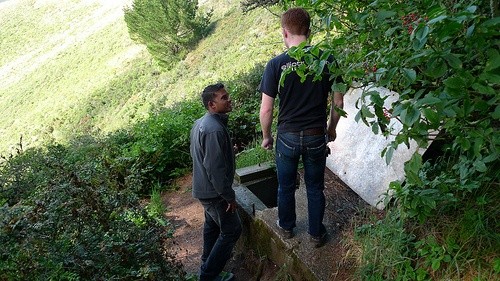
[260,7,344,248]
[190,83,242,281]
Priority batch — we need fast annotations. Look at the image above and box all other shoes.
[275,220,294,238]
[310,230,326,247]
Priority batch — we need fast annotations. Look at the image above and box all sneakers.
[217,271,234,281]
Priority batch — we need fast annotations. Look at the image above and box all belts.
[295,127,325,136]
